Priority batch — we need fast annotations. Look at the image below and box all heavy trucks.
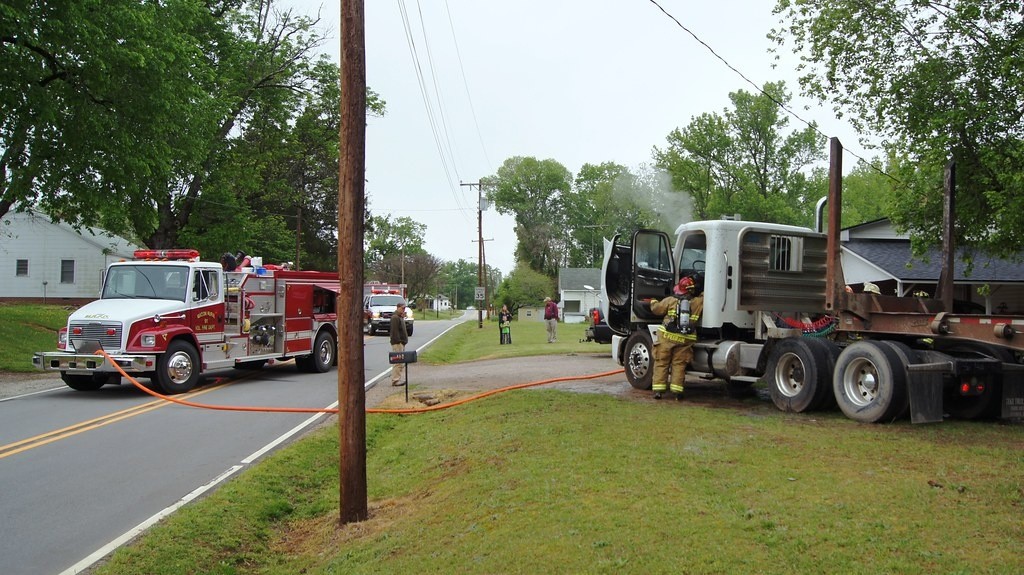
[583,195,1024,425]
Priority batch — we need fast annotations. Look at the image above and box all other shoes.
[675,394,683,400]
[652,392,662,399]
[392,380,406,385]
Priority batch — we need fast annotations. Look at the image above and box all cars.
[362,290,416,336]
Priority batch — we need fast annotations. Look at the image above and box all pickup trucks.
[579,307,616,345]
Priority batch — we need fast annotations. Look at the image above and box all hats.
[674,277,694,295]
[544,297,552,302]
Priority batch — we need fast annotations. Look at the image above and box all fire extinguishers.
[680,299,693,334]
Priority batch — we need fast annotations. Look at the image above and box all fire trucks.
[32,248,340,396]
[364,283,408,299]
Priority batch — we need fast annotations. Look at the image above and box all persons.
[499,305,513,344]
[643,277,704,401]
[544,297,558,343]
[390,302,408,386]
